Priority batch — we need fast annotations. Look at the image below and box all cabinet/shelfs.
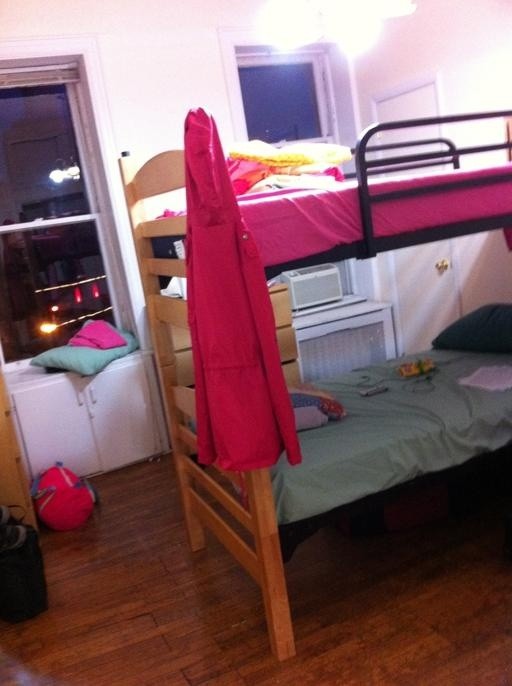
[11,361,163,486]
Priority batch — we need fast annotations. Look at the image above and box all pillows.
[431,303,512,355]
[30,320,139,376]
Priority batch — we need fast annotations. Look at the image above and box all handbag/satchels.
[0,505,49,623]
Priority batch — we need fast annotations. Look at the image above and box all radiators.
[294,307,396,384]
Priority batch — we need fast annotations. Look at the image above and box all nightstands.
[143,283,301,452]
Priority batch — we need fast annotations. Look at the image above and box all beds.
[116,109,512,660]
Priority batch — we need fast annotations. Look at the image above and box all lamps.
[49,155,80,183]
